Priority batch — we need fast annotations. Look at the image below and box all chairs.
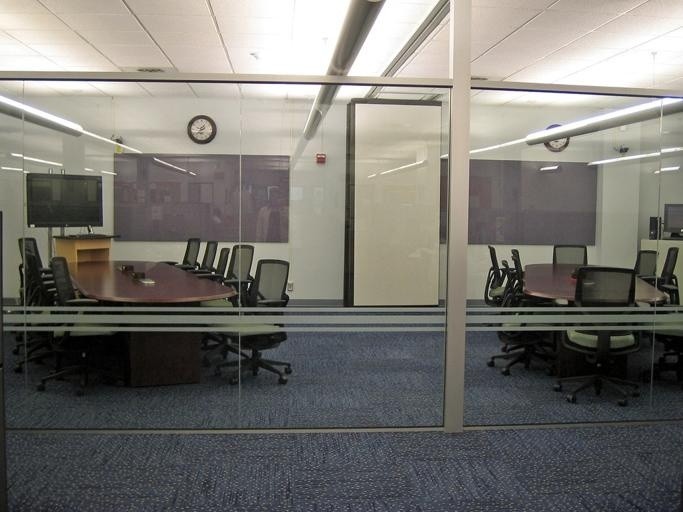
[480,241,683,411]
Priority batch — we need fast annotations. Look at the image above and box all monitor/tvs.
[663,203,683,239]
[27,172,102,227]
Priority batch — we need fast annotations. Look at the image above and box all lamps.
[613,136,633,156]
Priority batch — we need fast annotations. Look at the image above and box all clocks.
[542,123,572,154]
[188,115,218,148]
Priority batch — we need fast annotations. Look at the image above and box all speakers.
[648,216,662,239]
